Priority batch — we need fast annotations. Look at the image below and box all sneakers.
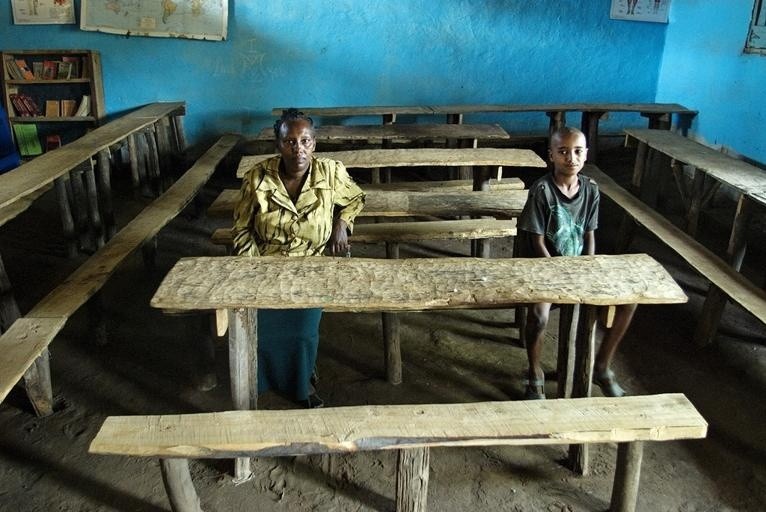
[300,365,324,409]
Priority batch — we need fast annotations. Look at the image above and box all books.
[5,55,91,157]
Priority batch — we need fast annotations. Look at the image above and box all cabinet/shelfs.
[1,50,106,163]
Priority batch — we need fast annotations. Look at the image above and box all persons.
[514,125,639,401]
[227,104,365,408]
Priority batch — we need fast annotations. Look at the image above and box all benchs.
[212,104,765,386]
[1,131,240,420]
[88,394,708,510]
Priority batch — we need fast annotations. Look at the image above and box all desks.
[2,103,187,329]
[150,254,689,483]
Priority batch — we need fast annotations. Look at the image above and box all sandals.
[593,368,625,397]
[527,368,545,400]
